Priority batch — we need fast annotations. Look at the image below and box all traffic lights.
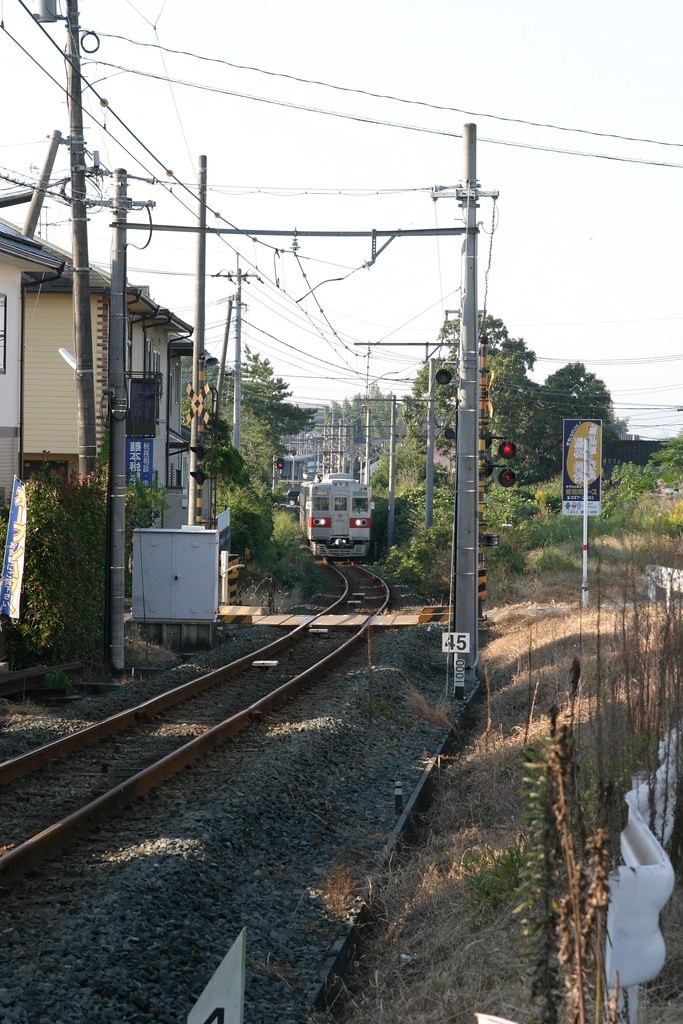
[445,429,455,440]
[435,369,452,385]
[190,444,207,460]
[499,440,517,460]
[276,458,285,469]
[498,467,516,487]
[190,470,207,485]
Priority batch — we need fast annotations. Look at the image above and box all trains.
[298,473,375,558]
